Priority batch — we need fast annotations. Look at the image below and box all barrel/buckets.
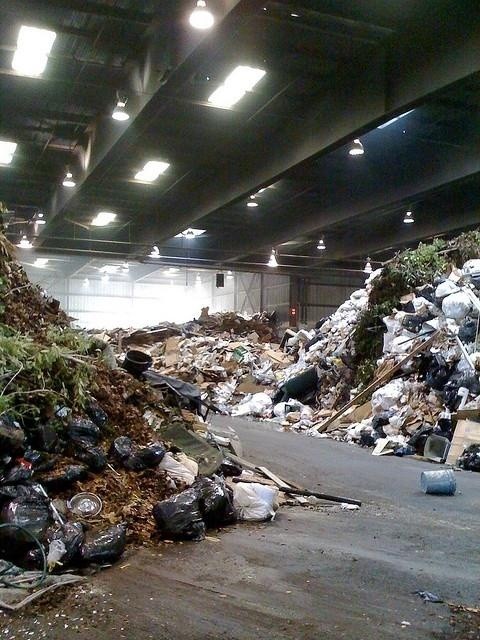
[121,350,154,380]
[420,469,457,496]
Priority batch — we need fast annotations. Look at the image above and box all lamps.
[186,1,215,31]
[247,193,259,209]
[151,242,160,257]
[19,161,79,247]
[109,96,130,122]
[262,205,421,271]
[346,137,367,157]
